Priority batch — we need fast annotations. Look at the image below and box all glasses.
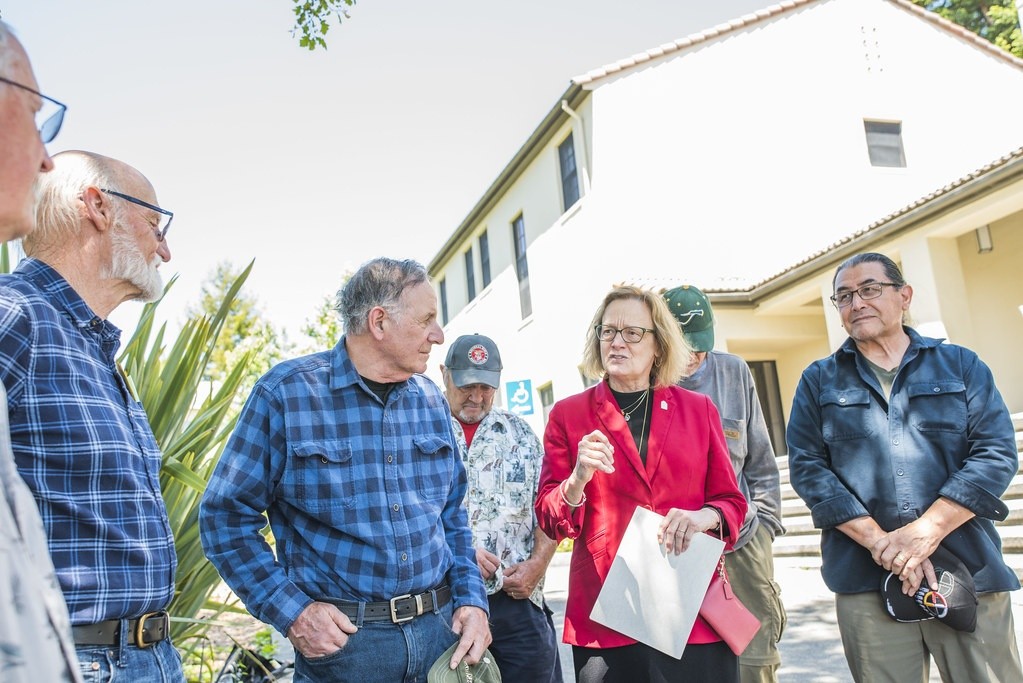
[830,281,902,310]
[81,188,174,241]
[0,76,67,144]
[595,324,655,344]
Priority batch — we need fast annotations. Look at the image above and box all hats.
[662,285,715,352]
[427,641,502,683]
[445,332,503,389]
[881,544,980,633]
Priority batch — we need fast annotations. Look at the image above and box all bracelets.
[560,479,586,507]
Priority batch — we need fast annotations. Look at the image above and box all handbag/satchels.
[698,507,762,656]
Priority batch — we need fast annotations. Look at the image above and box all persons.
[784,253,1023,683]
[0,22,183,683]
[534,284,748,683]
[199,258,493,683]
[664,284,788,682]
[439,333,565,683]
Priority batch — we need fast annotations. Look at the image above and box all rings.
[677,529,685,533]
[511,591,517,598]
[896,554,908,563]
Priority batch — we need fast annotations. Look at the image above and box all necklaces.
[622,389,649,453]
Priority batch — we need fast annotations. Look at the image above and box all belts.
[72,610,170,648]
[317,580,451,623]
